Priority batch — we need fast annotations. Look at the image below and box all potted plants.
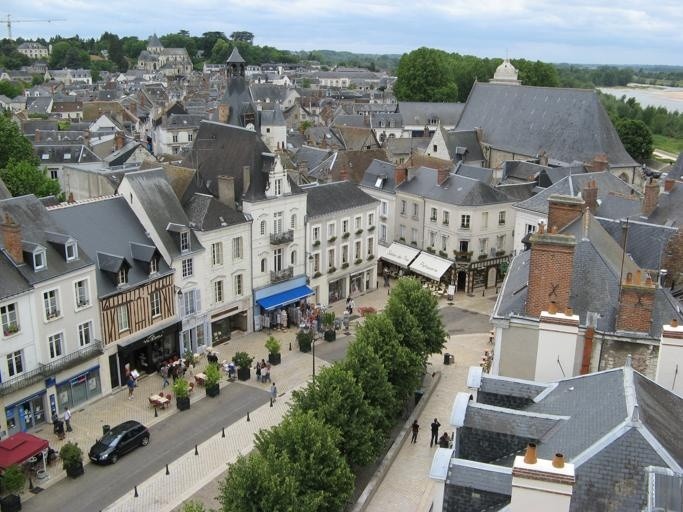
[295,329,313,353]
[202,361,223,397]
[322,311,335,341]
[230,351,255,380]
[263,335,282,365]
[57,441,84,478]
[0,463,27,512]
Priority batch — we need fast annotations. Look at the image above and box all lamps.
[304,251,314,263]
[173,285,182,300]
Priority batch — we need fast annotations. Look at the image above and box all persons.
[252,358,271,383]
[270,381,276,403]
[53,418,65,441]
[211,353,218,364]
[438,432,449,446]
[207,351,212,364]
[51,410,58,433]
[126,374,134,400]
[345,294,350,310]
[347,297,355,314]
[62,406,72,432]
[159,355,195,391]
[411,418,419,443]
[429,418,441,447]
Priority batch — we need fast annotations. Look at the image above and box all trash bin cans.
[443,353,454,365]
[103,425,110,436]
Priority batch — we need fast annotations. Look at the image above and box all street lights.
[312,336,321,387]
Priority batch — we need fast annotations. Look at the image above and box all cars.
[87,419,150,464]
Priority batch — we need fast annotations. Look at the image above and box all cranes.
[0,11,69,45]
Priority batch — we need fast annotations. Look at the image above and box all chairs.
[146,355,208,411]
